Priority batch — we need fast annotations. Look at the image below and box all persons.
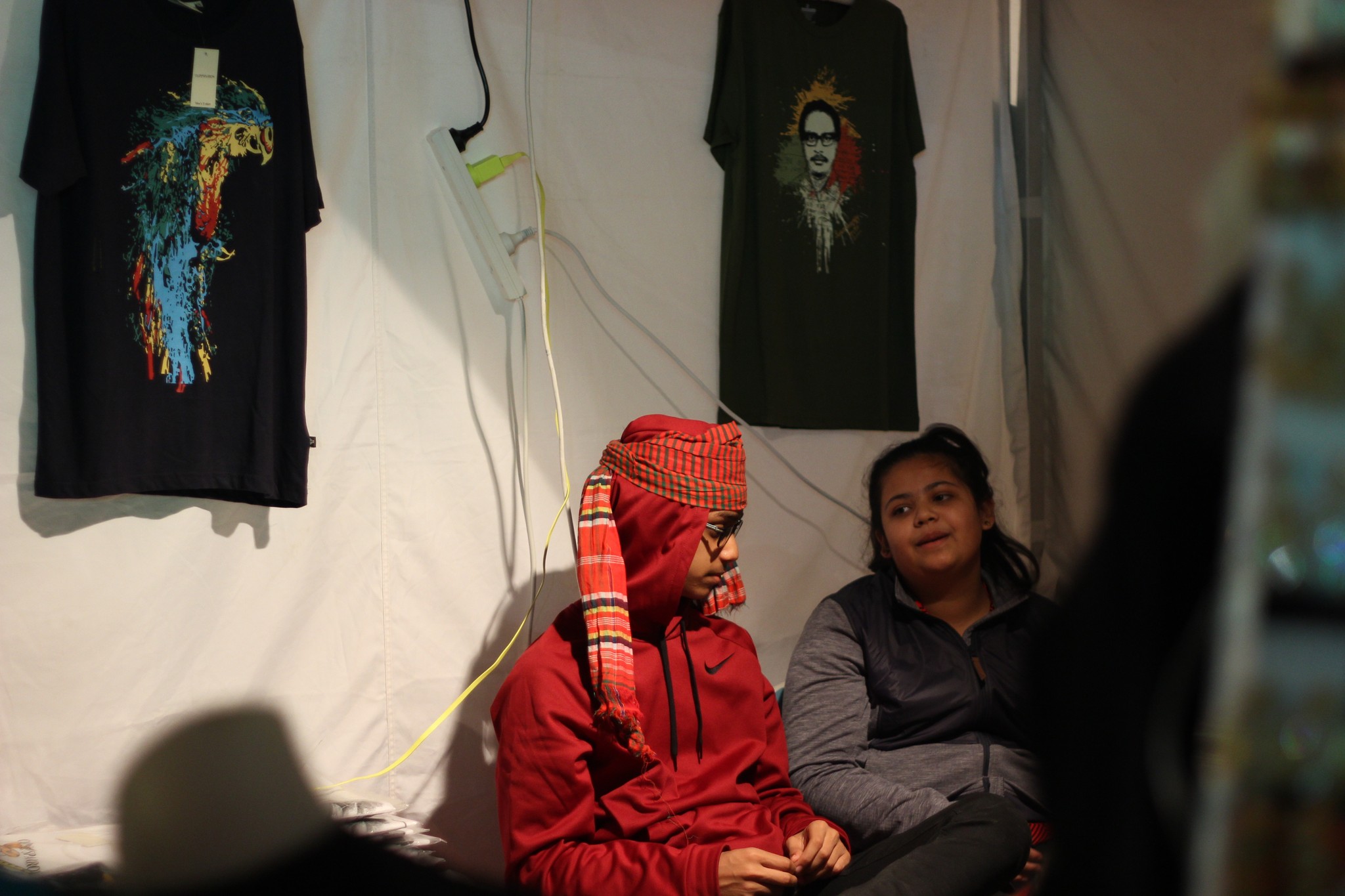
[488,414,855,896]
[780,422,1060,895]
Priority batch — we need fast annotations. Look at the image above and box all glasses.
[705,518,743,547]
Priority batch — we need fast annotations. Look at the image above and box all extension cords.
[425,125,527,302]
